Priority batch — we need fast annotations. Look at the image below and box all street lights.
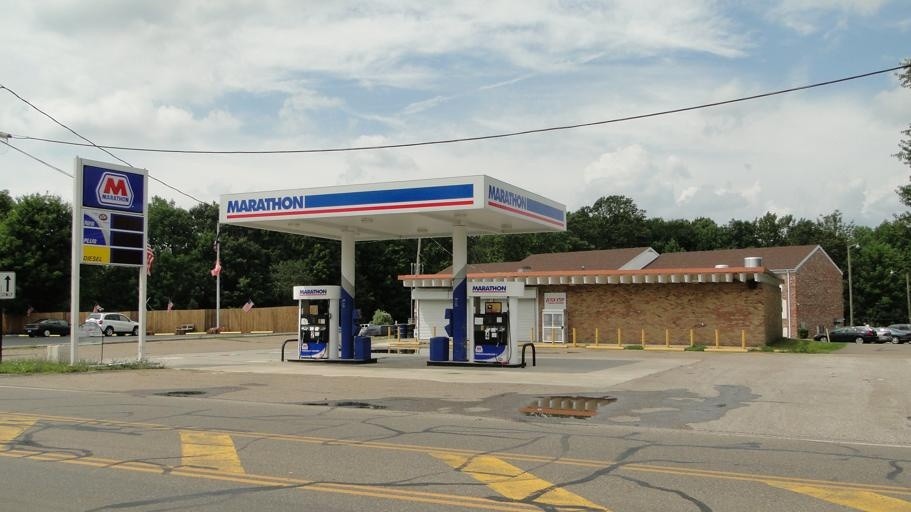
[890,270,911,322]
[847,243,860,325]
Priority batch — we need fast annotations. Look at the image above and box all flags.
[168,300,173,312]
[27,307,33,317]
[242,299,254,313]
[147,239,154,276]
[93,303,99,313]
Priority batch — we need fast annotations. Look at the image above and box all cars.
[85,312,139,337]
[360,324,370,329]
[813,323,911,344]
[23,318,71,337]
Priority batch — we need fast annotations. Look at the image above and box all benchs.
[176,323,195,335]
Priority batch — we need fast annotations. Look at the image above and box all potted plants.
[366,307,396,336]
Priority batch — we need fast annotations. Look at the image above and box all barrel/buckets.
[430,337,450,361]
[355,335,371,359]
